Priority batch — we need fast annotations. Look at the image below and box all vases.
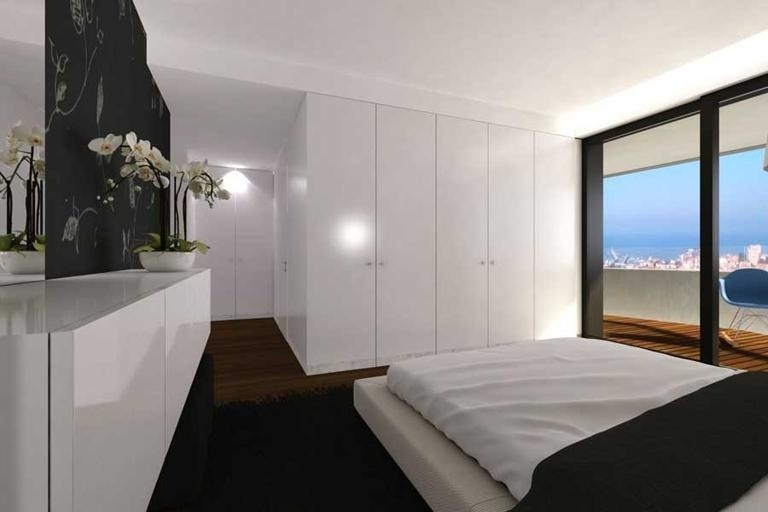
[131,244,200,275]
[0,248,47,277]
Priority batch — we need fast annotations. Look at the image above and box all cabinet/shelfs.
[308,92,436,376]
[534,130,584,342]
[271,96,308,378]
[435,112,534,352]
[1,261,220,512]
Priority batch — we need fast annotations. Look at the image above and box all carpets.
[194,369,438,512]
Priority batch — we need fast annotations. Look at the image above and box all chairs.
[716,266,768,342]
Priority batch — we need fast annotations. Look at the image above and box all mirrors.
[0,1,50,290]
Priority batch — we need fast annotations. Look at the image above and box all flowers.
[77,123,234,256]
[0,114,50,256]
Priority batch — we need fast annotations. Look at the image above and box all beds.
[348,333,768,512]
